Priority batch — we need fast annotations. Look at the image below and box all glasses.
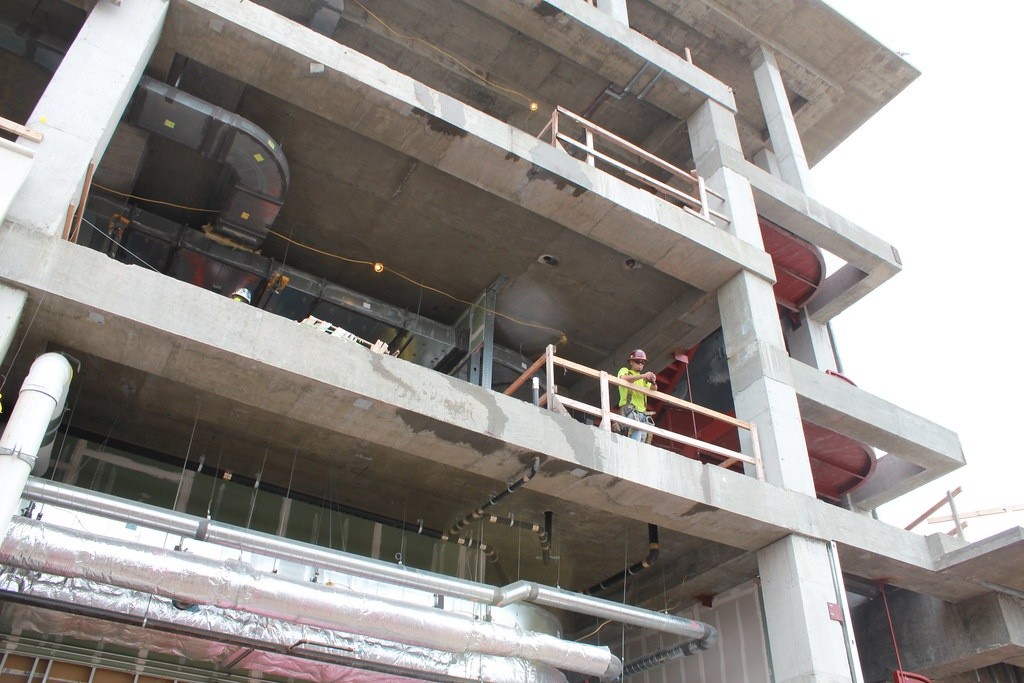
[628,349,648,363]
[631,359,645,365]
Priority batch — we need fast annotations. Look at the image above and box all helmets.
[232,288,252,303]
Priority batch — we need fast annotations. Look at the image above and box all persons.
[617,349,658,443]
[231,288,251,304]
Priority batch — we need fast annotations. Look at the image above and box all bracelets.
[652,382,655,385]
